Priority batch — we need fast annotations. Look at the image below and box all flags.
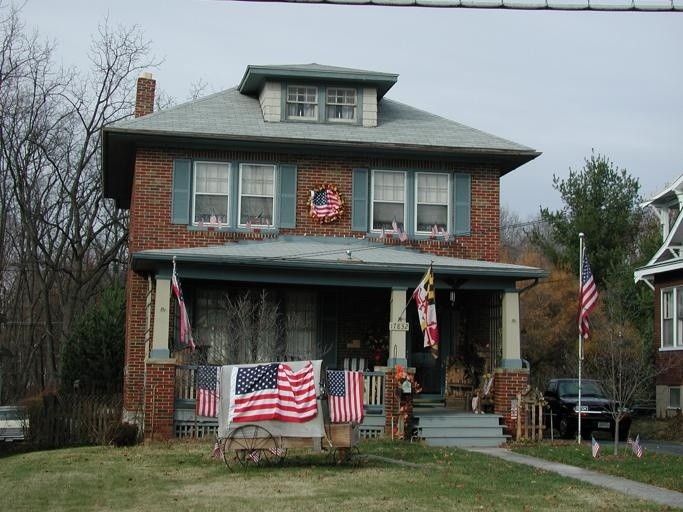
[380,216,451,245]
[627,437,635,448]
[195,364,220,417]
[632,436,641,456]
[170,266,197,353]
[574,241,600,344]
[248,450,262,462]
[589,436,600,457]
[211,443,220,457]
[325,369,365,423]
[227,360,318,423]
[267,447,284,456]
[196,211,272,234]
[412,264,441,359]
[308,187,341,217]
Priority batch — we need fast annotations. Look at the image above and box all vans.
[0,405,31,441]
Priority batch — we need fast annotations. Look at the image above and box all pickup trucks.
[531,378,632,441]
